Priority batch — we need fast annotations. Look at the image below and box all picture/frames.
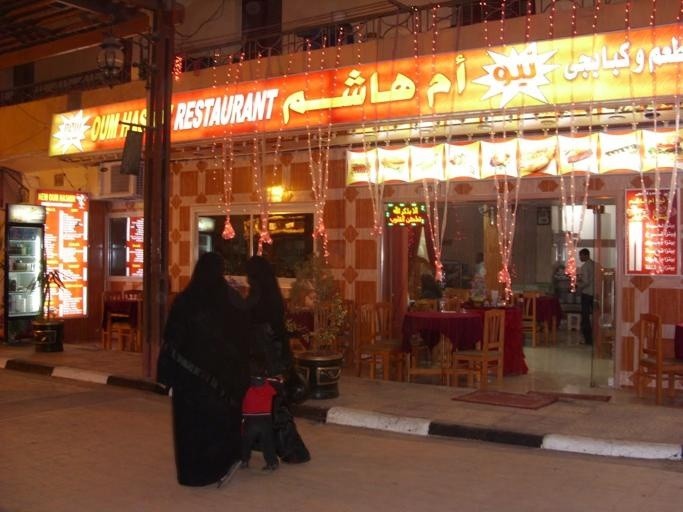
[535,206,552,225]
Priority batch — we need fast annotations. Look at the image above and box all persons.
[241,255,310,464]
[154,251,247,489]
[575,247,604,345]
[240,367,280,472]
[470,251,487,287]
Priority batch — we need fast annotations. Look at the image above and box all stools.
[568,311,582,331]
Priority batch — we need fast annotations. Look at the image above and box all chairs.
[635,311,682,404]
[102,285,146,353]
[284,288,557,390]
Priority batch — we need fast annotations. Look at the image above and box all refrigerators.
[0,201,46,344]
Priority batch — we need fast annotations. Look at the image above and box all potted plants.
[27,269,66,352]
[279,236,348,401]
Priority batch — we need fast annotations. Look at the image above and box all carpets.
[449,387,558,410]
[523,389,612,404]
